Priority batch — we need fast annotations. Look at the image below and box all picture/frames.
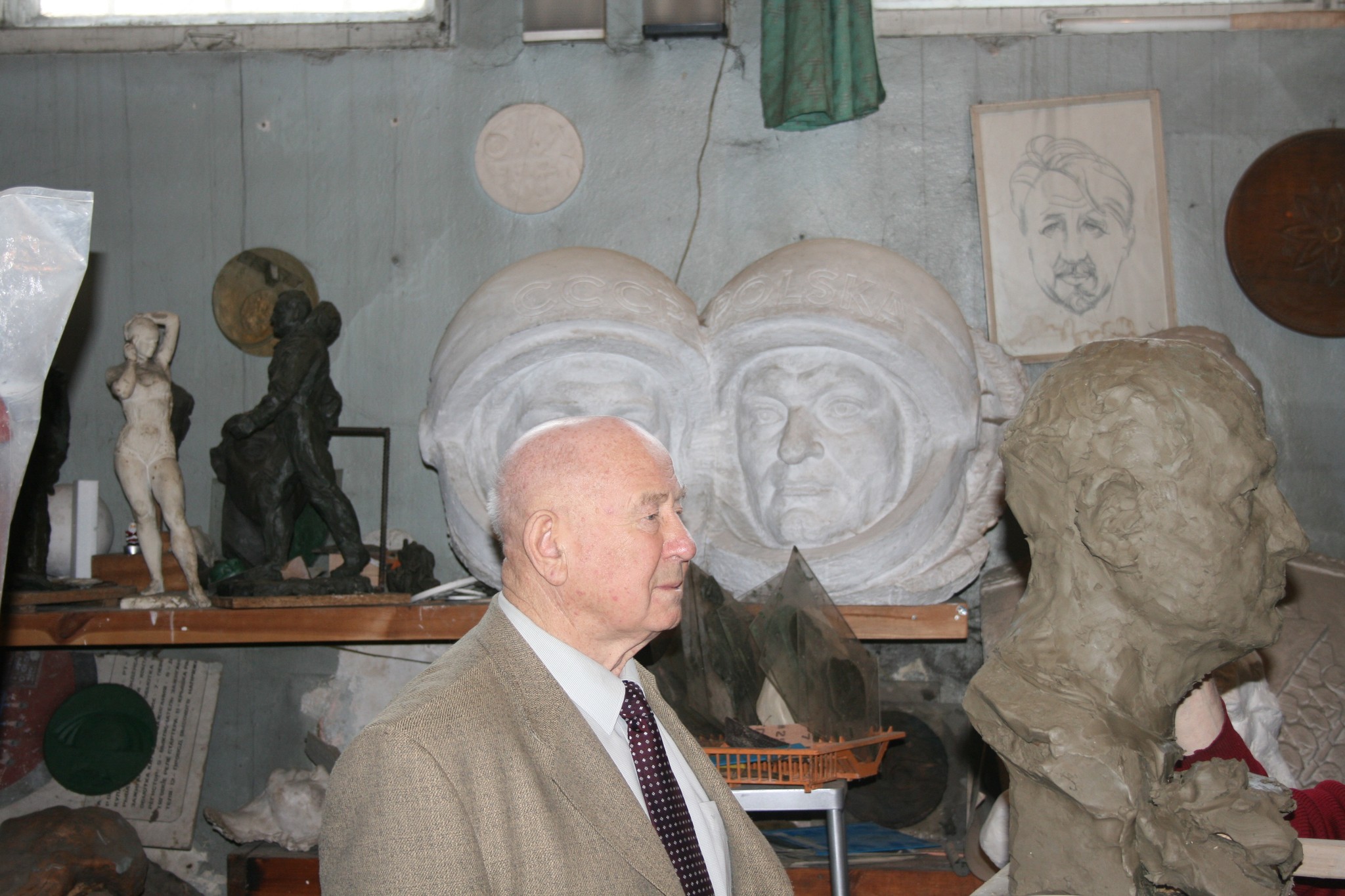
[329,426,390,593]
[969,90,1179,363]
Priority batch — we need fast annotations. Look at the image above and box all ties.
[621,679,715,896]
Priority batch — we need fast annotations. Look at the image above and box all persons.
[318,414,796,896]
[963,328,1309,819]
[103,310,213,607]
[222,288,372,580]
[418,237,1029,608]
